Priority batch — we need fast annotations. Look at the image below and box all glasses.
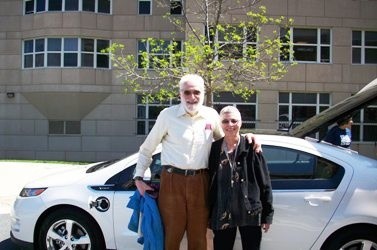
[222,119,240,124]
[184,90,201,96]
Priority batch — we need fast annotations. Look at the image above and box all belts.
[164,165,208,176]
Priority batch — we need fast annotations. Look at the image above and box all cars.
[11,133,377,250]
